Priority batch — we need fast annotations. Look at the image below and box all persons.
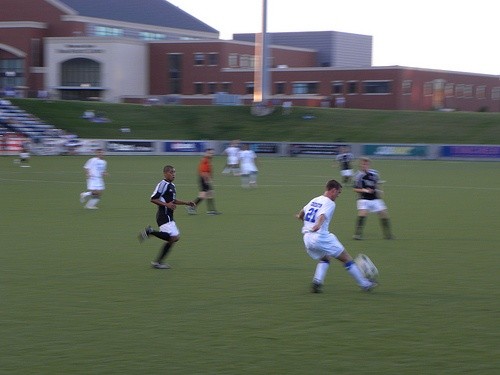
[338,147,355,182]
[138,165,196,268]
[223,141,259,190]
[296,180,377,293]
[352,157,392,240]
[186,148,222,216]
[80,149,109,209]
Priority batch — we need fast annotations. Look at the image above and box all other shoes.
[80,191,86,203]
[206,209,222,215]
[311,281,322,293]
[365,281,379,291]
[185,206,197,215]
[384,233,396,238]
[138,226,154,240]
[84,205,99,210]
[352,233,362,239]
[151,261,169,269]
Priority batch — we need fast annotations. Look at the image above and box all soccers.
[354,253,380,282]
